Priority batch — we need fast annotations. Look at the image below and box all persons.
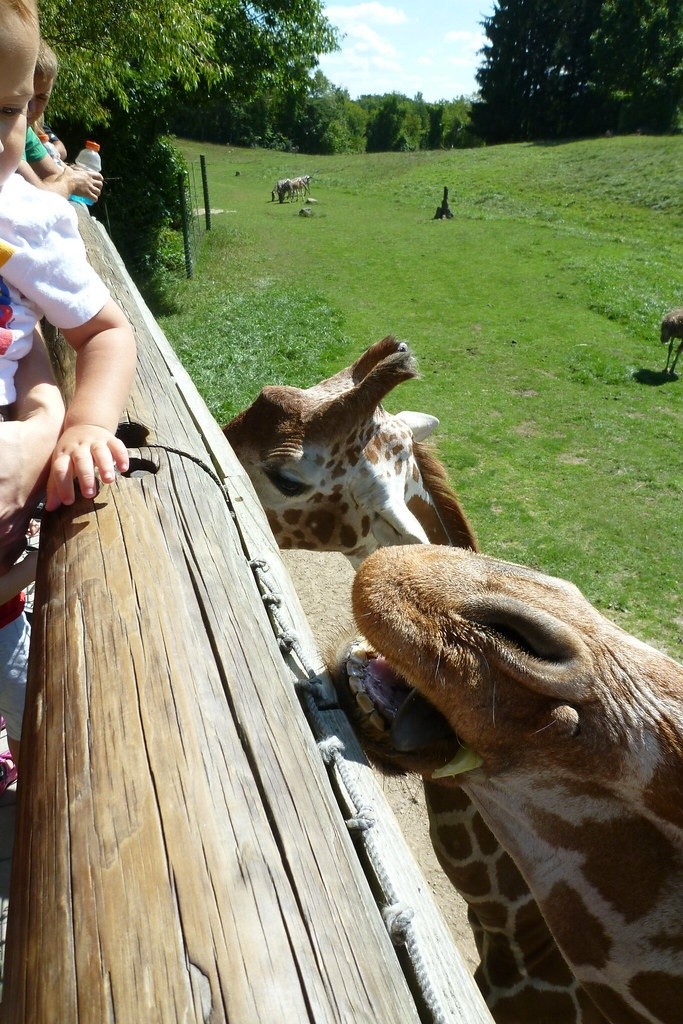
[0,1,137,796]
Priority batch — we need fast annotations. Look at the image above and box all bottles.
[38,134,61,167]
[68,140,102,206]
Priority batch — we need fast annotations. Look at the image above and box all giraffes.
[324,543,682,1024]
[221,334,479,572]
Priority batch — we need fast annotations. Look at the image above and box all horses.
[271,175,312,204]
